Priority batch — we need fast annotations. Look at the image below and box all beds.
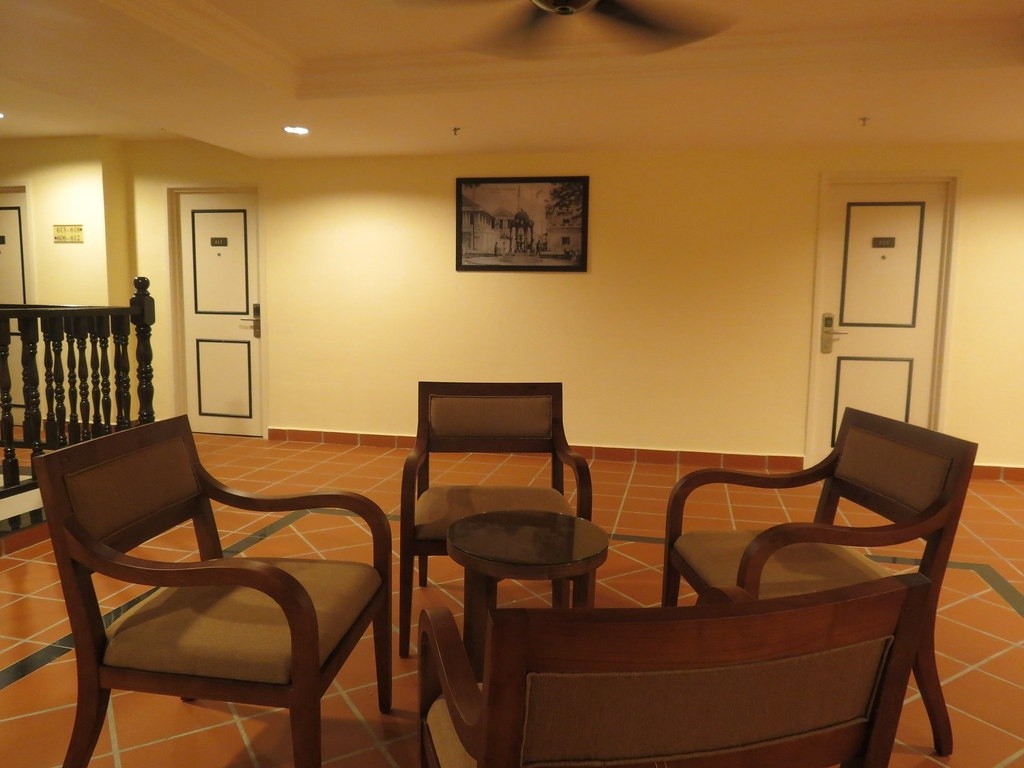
[418,573,931,768]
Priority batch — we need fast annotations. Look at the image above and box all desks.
[447,510,608,683]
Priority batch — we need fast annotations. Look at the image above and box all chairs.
[663,407,978,755]
[399,381,592,658]
[34,414,392,768]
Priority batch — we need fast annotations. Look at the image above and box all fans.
[467,0,739,57]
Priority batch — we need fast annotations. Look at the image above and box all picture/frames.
[455,175,589,271]
